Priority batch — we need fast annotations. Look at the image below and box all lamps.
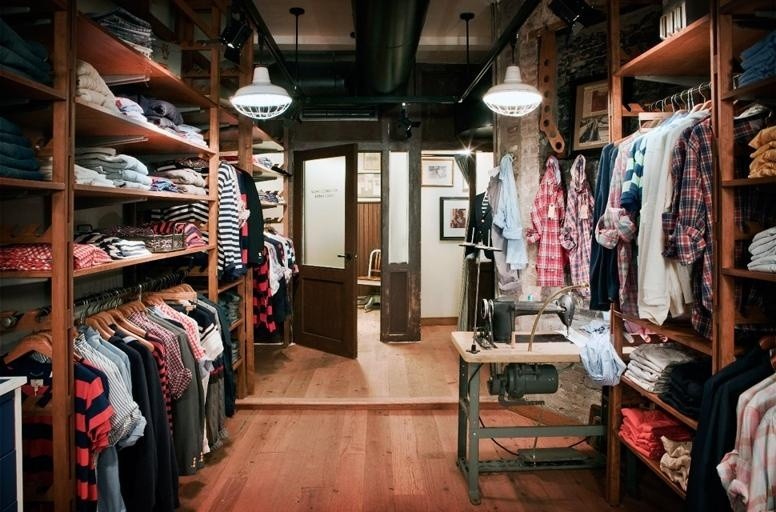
[482,37,543,118]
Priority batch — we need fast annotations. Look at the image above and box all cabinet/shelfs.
[717,0,776,511]
[0,0,288,511]
[604,0,716,507]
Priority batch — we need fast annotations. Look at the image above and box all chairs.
[357,249,381,313]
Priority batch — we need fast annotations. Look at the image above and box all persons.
[451,210,465,228]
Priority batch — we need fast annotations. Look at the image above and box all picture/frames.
[357,152,381,174]
[421,157,454,187]
[568,74,609,159]
[357,174,382,204]
[440,197,469,240]
